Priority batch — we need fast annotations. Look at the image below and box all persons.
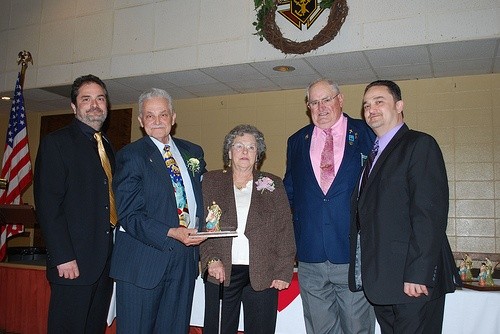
[33,74,117,334]
[348,80,462,334]
[283,79,377,334]
[110,87,208,334]
[460,253,473,280]
[200,125,297,334]
[478,257,496,286]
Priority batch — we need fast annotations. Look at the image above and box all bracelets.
[207,258,221,266]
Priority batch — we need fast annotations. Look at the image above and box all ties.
[162,145,191,228]
[320,128,335,195]
[93,132,117,226]
[356,140,382,233]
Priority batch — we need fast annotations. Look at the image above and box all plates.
[190,231,238,237]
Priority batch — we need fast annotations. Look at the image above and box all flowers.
[186,157,201,177]
[254,176,277,195]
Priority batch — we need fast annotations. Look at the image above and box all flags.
[0,76,33,262]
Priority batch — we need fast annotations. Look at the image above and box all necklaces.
[234,173,250,190]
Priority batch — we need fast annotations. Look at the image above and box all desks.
[0,262,117,334]
[104,269,500,334]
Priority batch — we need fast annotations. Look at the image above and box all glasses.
[231,142,257,153]
[309,94,339,109]
